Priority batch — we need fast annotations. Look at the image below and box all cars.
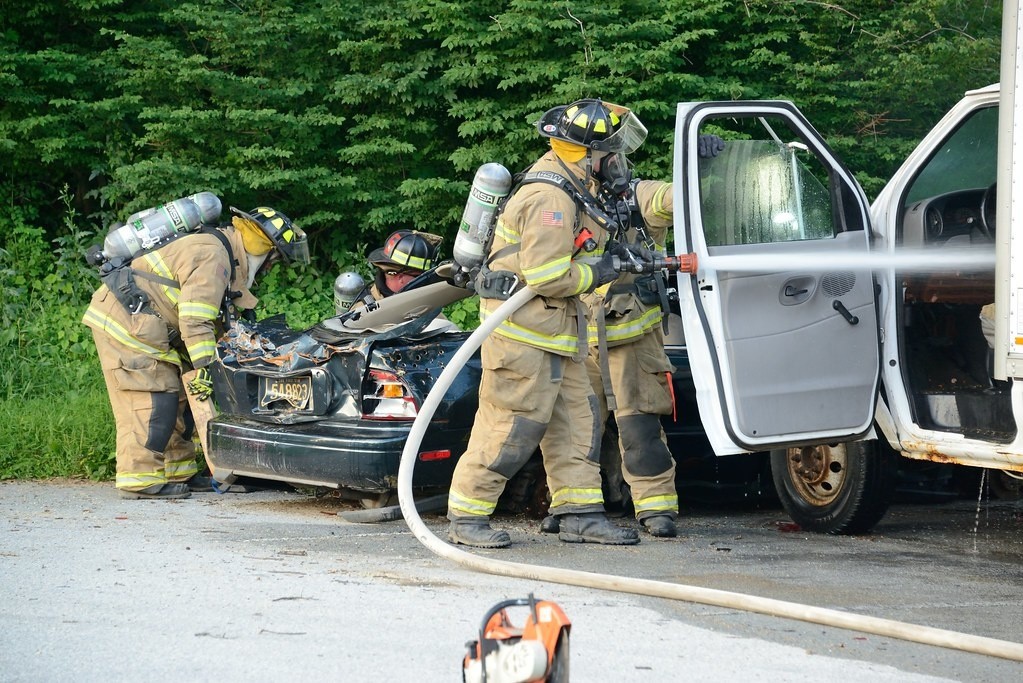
[203,312,779,512]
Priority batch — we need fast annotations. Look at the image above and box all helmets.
[243,203,311,269]
[368,228,439,275]
[536,97,650,154]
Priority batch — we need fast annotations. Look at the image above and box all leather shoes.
[539,513,560,532]
[606,501,625,518]
[183,476,215,491]
[644,515,678,538]
[447,521,512,548]
[120,480,192,500]
[557,511,641,545]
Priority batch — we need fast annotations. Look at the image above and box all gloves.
[610,243,662,266]
[697,133,724,176]
[584,250,620,293]
[186,367,214,402]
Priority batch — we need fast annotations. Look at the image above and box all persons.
[81,207,297,500]
[349,228,448,322]
[447,101,724,549]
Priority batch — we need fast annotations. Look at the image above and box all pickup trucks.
[672,80,1023,538]
